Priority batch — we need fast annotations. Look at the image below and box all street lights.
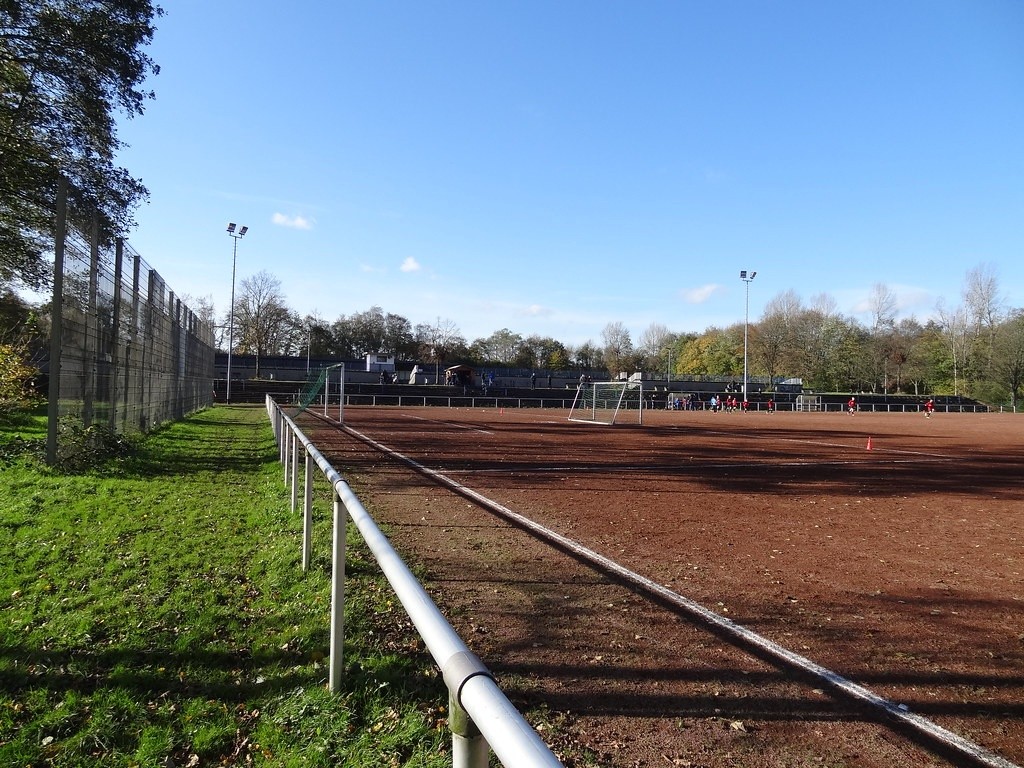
[226,222,249,405]
[741,270,756,402]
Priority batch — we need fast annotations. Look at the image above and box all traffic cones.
[864,436,873,451]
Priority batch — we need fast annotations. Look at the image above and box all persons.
[768,398,773,413]
[391,372,398,384]
[924,399,934,419]
[847,397,855,417]
[481,370,494,386]
[378,373,385,384]
[531,373,536,390]
[743,399,749,414]
[668,394,699,411]
[548,375,552,390]
[710,395,738,414]
[578,374,592,389]
[726,381,741,392]
